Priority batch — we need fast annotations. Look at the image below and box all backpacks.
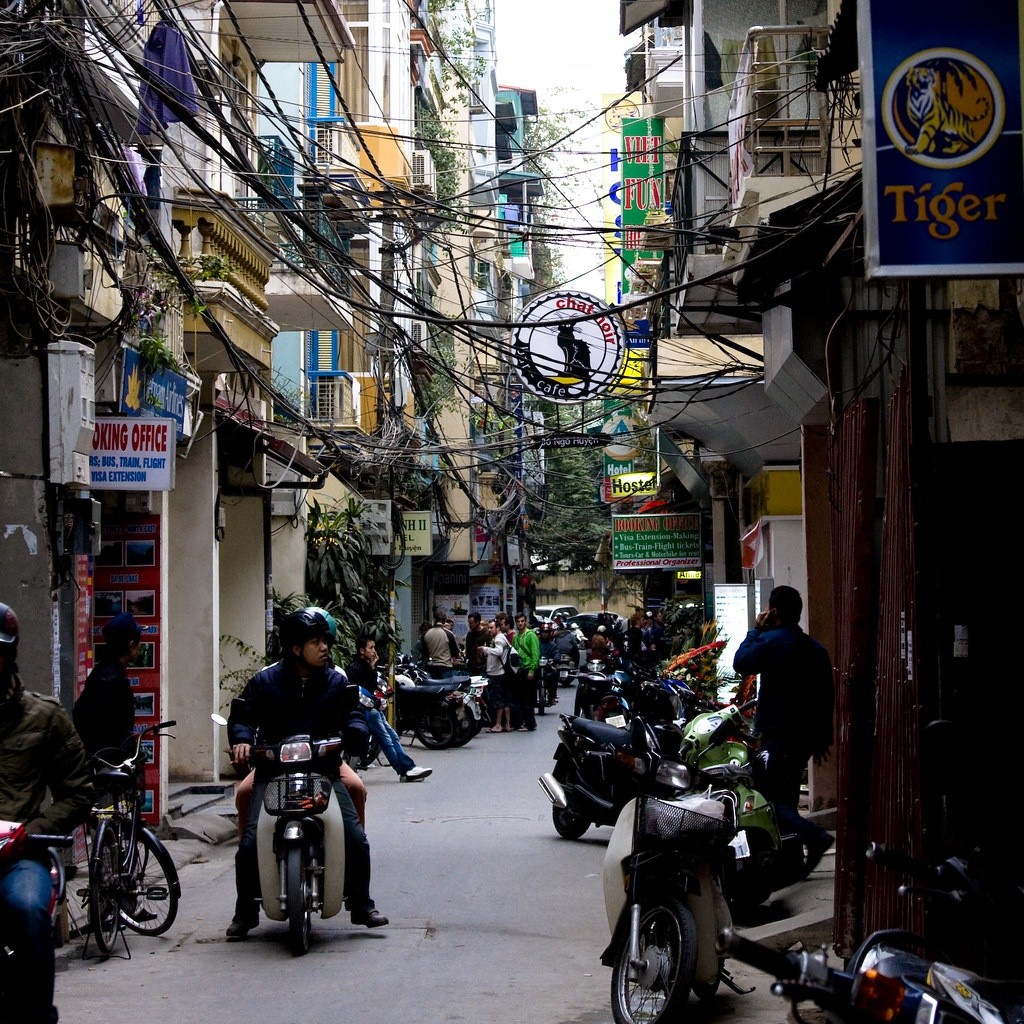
[492,638,520,678]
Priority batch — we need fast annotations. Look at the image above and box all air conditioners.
[314,128,360,173]
[411,150,438,204]
[409,319,429,353]
[315,374,355,425]
[470,80,483,114]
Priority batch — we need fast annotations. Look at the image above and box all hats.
[102,612,150,643]
[597,625,606,632]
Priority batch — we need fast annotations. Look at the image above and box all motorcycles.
[344,654,491,764]
[211,713,372,955]
[713,718,1023,1024]
[536,644,795,1024]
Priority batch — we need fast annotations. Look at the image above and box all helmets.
[279,611,330,650]
[540,623,551,639]
[303,607,336,645]
[0,602,20,645]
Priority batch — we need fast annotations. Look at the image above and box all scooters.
[0,820,76,937]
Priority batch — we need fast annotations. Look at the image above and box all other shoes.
[226,916,260,937]
[132,908,158,922]
[803,833,835,878]
[351,907,389,928]
[400,768,433,782]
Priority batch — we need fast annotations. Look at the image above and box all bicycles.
[79,721,182,963]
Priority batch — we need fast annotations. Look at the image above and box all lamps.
[232,41,242,67]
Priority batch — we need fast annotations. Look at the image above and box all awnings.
[735,171,863,304]
[815,0,858,89]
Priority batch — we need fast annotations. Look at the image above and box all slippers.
[485,728,502,733]
[502,726,511,732]
[518,726,537,731]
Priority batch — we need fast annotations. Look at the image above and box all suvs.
[535,604,580,623]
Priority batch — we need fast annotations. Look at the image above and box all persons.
[466,612,539,733]
[414,610,460,679]
[0,602,95,1024]
[530,612,586,709]
[345,635,432,782]
[733,586,835,885]
[226,606,388,939]
[591,606,669,676]
[73,611,157,931]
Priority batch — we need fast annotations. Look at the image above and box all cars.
[564,612,627,636]
